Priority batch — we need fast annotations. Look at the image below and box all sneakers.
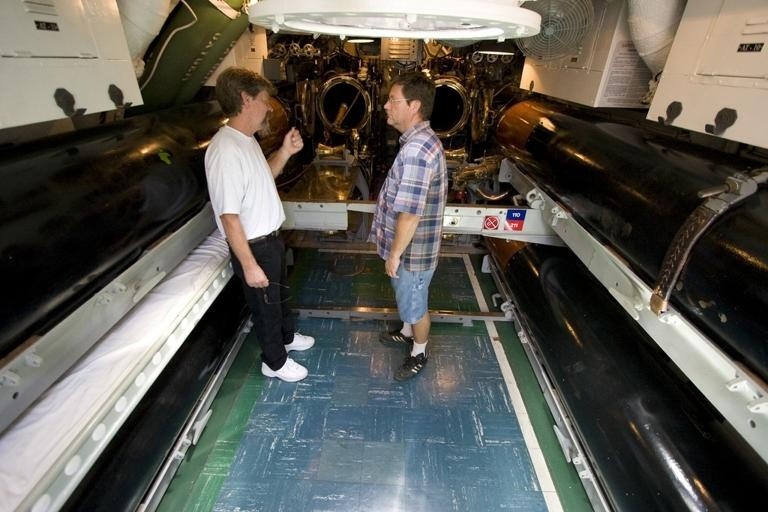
[394,351,428,381]
[379,329,414,346]
[261,357,308,382]
[283,333,315,353]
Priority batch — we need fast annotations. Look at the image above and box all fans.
[515,0,595,62]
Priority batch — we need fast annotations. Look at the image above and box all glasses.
[263,281,292,304]
[385,95,425,105]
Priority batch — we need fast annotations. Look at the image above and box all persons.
[205,67,315,383]
[367,72,448,382]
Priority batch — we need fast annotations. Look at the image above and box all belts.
[249,228,282,246]
